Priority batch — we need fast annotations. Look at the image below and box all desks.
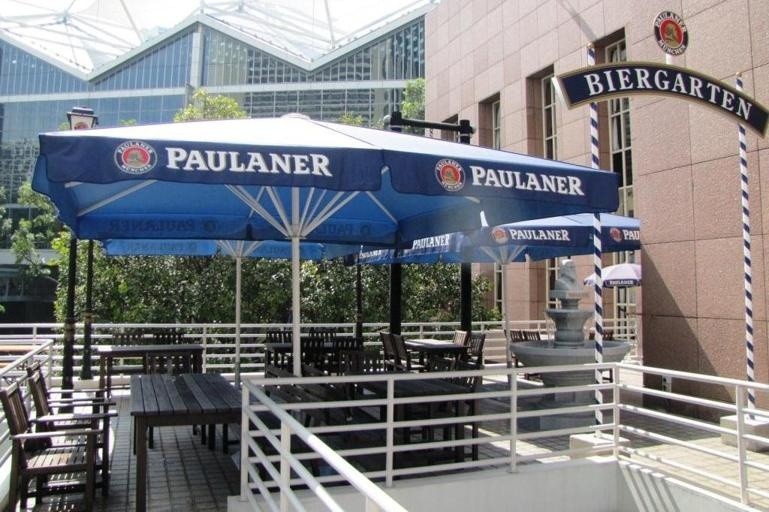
[97,343,205,398]
[129,373,240,512]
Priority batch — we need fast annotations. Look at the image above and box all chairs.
[266,326,614,473]
[0,361,119,512]
[107,333,148,397]
[151,331,182,373]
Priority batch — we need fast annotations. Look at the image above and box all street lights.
[61,102,101,415]
